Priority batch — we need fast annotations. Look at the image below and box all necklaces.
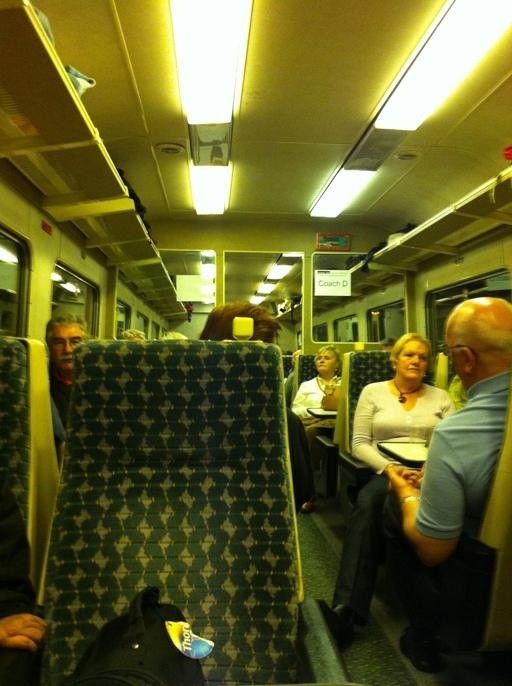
[394,381,421,403]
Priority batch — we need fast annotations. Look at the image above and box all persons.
[198,302,316,515]
[316,333,460,650]
[120,328,145,340]
[0,487,49,653]
[45,312,92,466]
[379,338,396,351]
[291,345,342,513]
[385,296,512,674]
[284,349,301,408]
[159,331,188,339]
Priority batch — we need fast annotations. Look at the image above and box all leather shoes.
[301,493,324,515]
[400,624,442,673]
[316,599,363,649]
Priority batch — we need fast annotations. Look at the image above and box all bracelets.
[399,496,420,507]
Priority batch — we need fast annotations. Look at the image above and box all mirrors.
[310,252,410,345]
[157,248,217,314]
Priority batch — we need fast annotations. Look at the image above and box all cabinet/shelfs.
[168,0,255,216]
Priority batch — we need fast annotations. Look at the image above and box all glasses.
[439,344,477,356]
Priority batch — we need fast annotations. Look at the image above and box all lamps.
[306,0,512,218]
[53,271,82,296]
[199,251,216,304]
[0,243,19,266]
[249,254,302,305]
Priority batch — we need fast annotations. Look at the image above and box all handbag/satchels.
[64,587,205,686]
